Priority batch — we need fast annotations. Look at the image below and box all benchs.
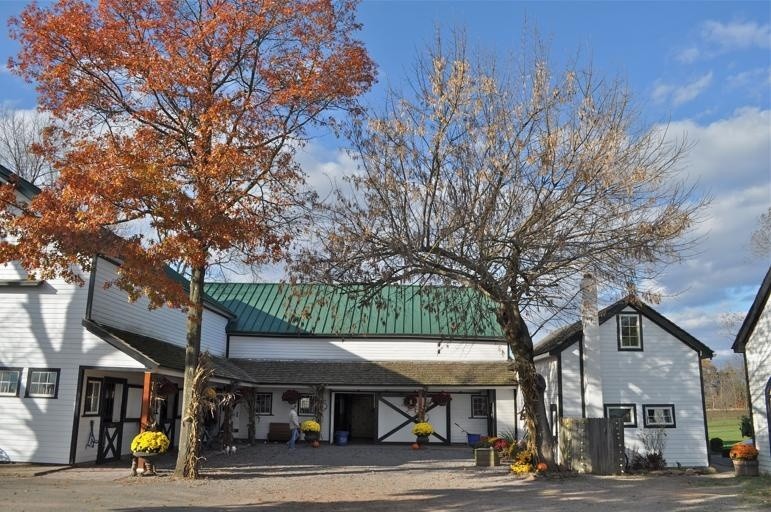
[267,423,301,443]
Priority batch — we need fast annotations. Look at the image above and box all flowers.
[411,423,433,436]
[300,420,321,433]
[728,443,759,461]
[131,431,171,455]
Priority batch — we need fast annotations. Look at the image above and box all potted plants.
[432,391,452,406]
[473,441,500,466]
[281,390,301,404]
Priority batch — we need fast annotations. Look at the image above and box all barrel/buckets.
[467,433,480,446]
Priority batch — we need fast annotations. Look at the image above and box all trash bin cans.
[467,434,479,446]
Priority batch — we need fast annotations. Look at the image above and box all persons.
[286,404,301,449]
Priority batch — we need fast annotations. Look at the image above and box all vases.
[132,452,159,476]
[732,455,759,476]
[415,433,429,446]
[303,431,318,443]
